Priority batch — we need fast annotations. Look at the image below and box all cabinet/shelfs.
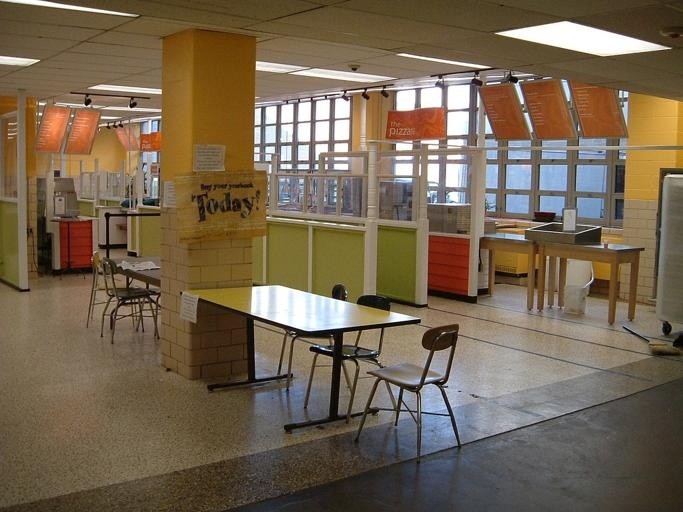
[96,206,127,248]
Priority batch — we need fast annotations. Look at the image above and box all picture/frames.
[519,78,578,140]
[569,81,628,138]
[479,84,532,142]
[65,108,101,156]
[35,103,70,156]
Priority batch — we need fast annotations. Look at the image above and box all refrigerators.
[491,221,540,279]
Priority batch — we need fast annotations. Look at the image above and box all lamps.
[104,121,125,129]
[69,92,151,108]
[338,84,393,101]
[430,67,543,89]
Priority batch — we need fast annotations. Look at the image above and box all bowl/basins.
[533,210,555,222]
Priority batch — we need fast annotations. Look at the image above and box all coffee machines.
[53,176,80,218]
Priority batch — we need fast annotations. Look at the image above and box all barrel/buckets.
[564,285,588,314]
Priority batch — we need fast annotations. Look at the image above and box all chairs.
[353,324,463,463]
[275,284,350,391]
[103,255,162,339]
[305,295,396,422]
[100,258,159,344]
[87,251,137,330]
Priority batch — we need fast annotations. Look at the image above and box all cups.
[603,240,608,248]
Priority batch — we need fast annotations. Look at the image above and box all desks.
[537,242,646,324]
[109,256,161,330]
[478,234,537,309]
[183,284,422,432]
